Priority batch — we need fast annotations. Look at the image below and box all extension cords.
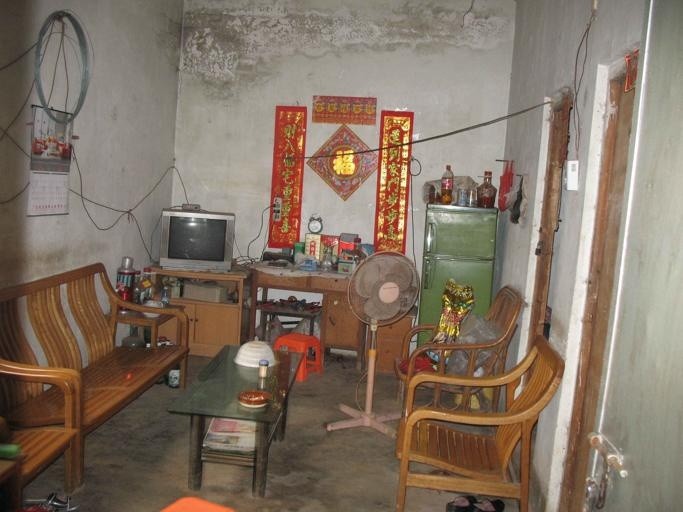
[273,197,282,222]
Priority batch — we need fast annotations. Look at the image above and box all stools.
[272,333,321,382]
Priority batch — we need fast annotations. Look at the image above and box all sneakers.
[446,495,505,512]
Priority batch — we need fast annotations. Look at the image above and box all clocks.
[309,217,323,233]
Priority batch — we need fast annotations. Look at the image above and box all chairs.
[395,285,565,511]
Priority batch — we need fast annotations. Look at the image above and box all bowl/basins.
[238,390,272,409]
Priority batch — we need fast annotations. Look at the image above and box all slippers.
[16,494,79,512]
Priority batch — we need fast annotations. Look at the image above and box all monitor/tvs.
[160,209,235,273]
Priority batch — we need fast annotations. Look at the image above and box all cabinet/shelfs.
[150,255,417,374]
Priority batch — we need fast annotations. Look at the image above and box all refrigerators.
[416,203,499,356]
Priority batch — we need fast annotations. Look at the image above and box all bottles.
[258,359,269,378]
[439,164,454,204]
[476,171,499,209]
[160,286,170,307]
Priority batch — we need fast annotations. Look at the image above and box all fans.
[325,250,421,438]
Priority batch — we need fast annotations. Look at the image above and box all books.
[201,417,257,457]
